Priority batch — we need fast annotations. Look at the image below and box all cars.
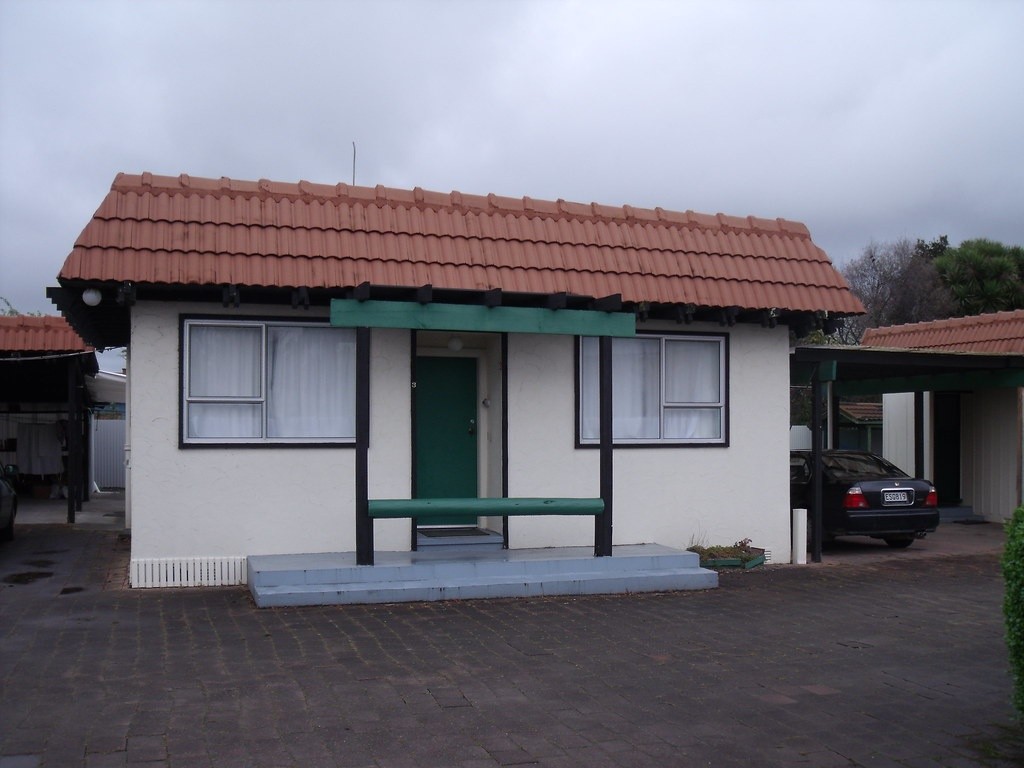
[790,451,941,553]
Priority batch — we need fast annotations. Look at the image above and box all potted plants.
[688,538,766,569]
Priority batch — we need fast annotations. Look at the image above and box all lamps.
[83,290,102,307]
